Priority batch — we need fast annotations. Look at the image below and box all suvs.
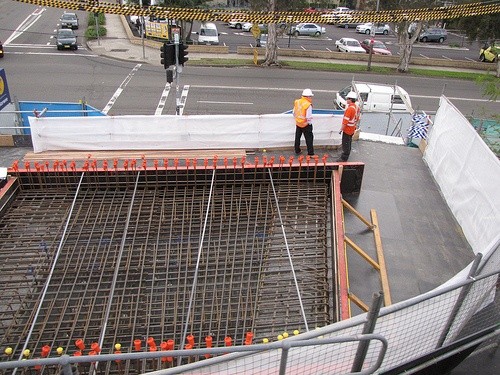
[419,28,448,43]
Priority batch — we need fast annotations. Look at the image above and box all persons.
[293,88,319,159]
[336,92,360,162]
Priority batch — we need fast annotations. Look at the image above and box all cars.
[335,38,366,53]
[337,23,364,29]
[336,8,349,12]
[242,23,268,32]
[356,22,391,35]
[61,13,79,29]
[360,38,392,55]
[130,14,150,24]
[228,21,249,29]
[479,45,500,62]
[0,40,4,57]
[304,7,336,12]
[279,23,326,37]
[54,29,78,50]
[153,18,175,23]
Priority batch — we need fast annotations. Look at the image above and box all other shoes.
[297,149,303,154]
[336,157,346,162]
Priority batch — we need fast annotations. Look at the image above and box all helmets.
[302,88,313,97]
[345,91,357,99]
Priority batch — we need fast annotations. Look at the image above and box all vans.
[332,80,412,111]
[196,23,220,45]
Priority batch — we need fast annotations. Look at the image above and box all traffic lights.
[179,41,188,64]
[160,42,176,69]
